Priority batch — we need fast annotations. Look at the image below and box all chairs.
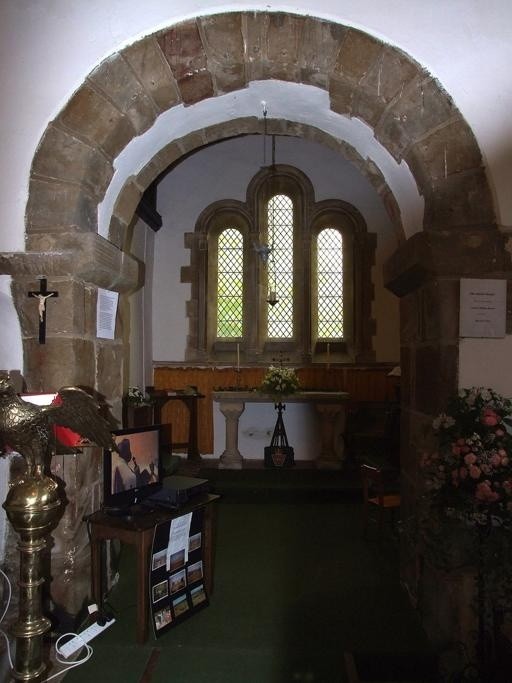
[362,464,400,556]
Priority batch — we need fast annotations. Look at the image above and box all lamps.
[265,133,278,306]
[255,100,272,269]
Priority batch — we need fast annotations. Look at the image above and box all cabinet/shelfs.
[398,276,511,683]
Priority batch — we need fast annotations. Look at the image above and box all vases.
[474,523,511,683]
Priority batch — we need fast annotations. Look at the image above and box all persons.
[31,291,55,322]
[132,456,142,487]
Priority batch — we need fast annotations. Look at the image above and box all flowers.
[251,364,304,401]
[416,386,512,532]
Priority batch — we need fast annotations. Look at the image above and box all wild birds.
[0,370,124,477]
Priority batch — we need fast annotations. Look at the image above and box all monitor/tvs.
[103,425,167,517]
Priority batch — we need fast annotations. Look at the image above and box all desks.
[122,390,205,462]
[210,388,352,472]
[82,489,221,644]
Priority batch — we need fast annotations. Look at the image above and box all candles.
[236,343,240,372]
[327,343,330,372]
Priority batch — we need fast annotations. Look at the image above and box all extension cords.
[58,616,115,659]
